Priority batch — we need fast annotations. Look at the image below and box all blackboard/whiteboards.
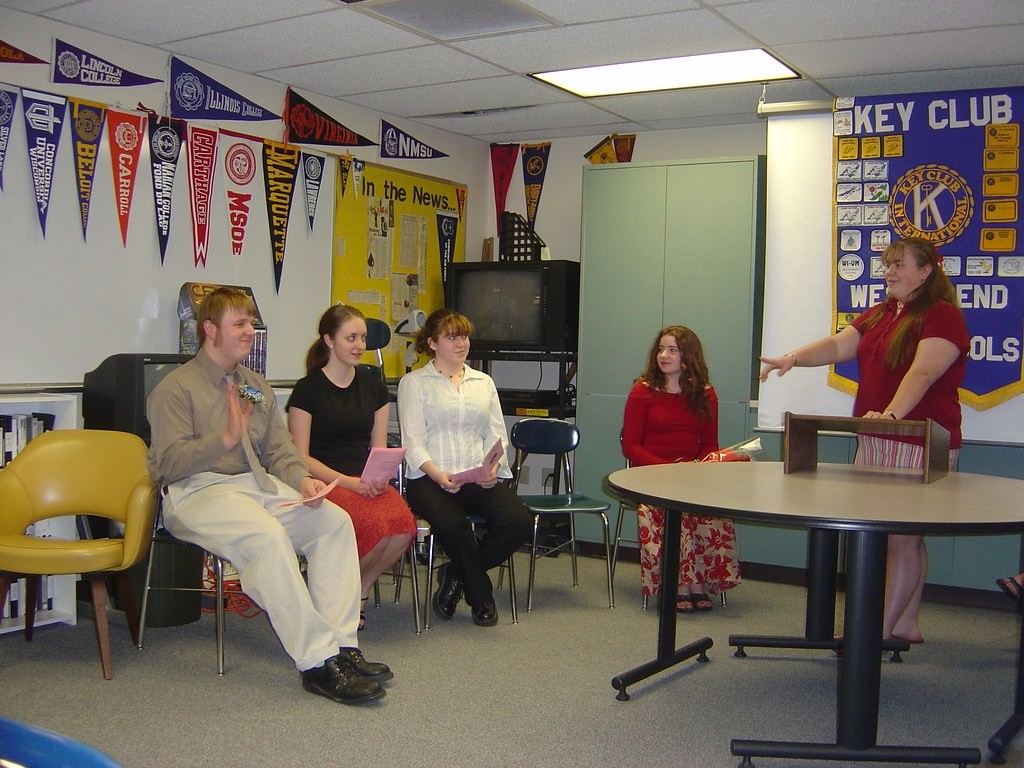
[0,82,337,394]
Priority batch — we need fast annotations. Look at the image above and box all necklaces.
[896,301,905,309]
[432,361,465,378]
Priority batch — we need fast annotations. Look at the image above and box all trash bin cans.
[0,716,122,768]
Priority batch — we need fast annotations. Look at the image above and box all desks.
[609,461,1024,768]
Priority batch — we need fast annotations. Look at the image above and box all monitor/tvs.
[447,259,579,354]
[82,354,196,449]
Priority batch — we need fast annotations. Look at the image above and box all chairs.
[0,318,726,768]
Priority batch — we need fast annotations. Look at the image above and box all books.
[2,517,53,619]
[0,413,56,469]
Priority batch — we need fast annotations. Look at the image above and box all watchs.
[883,410,897,420]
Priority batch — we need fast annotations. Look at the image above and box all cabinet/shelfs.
[465,349,576,557]
[0,394,80,634]
[572,156,1023,613]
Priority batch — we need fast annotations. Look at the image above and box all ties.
[223,371,280,495]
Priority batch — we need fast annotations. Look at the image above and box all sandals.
[691,591,713,612]
[676,594,694,613]
[356,596,369,629]
[996,576,1023,601]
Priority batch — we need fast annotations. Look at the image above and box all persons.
[284,303,418,630]
[145,288,393,706]
[995,573,1024,602]
[622,326,742,613]
[397,309,532,628]
[759,237,969,648]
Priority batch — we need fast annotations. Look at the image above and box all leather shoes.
[300,653,387,704]
[471,594,499,626]
[433,561,465,620]
[338,647,393,683]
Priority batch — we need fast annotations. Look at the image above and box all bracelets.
[784,352,797,366]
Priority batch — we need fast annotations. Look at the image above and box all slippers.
[890,633,925,644]
[833,631,887,656]
